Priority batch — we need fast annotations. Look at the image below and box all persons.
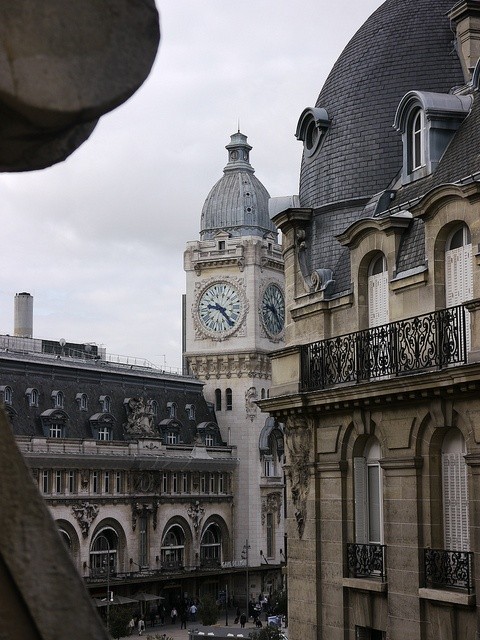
[170,607,177,624]
[160,607,166,625]
[239,613,247,628]
[235,606,241,622]
[248,593,268,627]
[189,603,197,621]
[128,613,146,637]
[180,611,188,631]
[148,608,156,627]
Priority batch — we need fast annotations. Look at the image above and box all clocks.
[257,275,285,343]
[191,274,249,340]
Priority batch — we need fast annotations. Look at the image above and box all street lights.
[101,546,115,635]
[240,539,253,622]
